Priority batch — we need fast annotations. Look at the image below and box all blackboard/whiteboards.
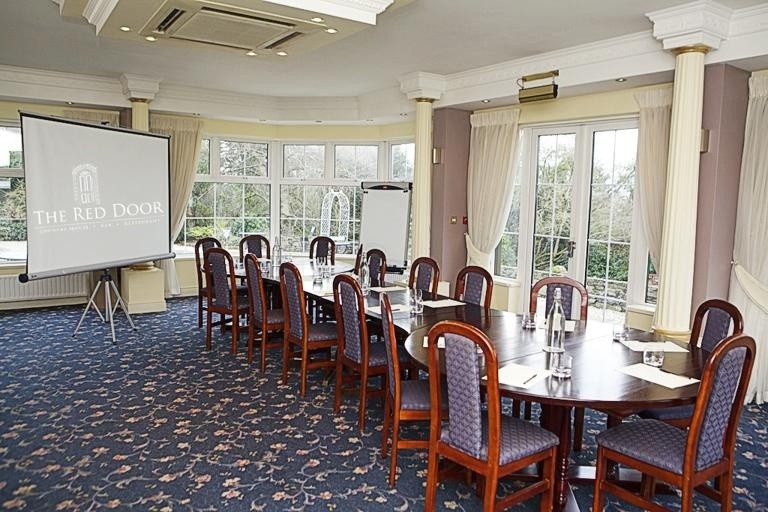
[359,181,412,274]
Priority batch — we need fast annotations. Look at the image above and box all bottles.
[545,288,566,351]
[360,253,370,290]
[272,235,280,266]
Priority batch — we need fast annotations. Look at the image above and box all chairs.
[377,292,474,490]
[245,253,310,373]
[333,274,419,429]
[305,236,335,314]
[512,277,588,453]
[239,234,279,320]
[453,265,493,309]
[594,336,757,512]
[195,237,245,335]
[366,249,387,342]
[280,263,355,398]
[408,257,440,294]
[637,299,743,430]
[204,248,270,354]
[424,319,559,510]
[316,244,363,322]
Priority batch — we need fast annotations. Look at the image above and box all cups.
[522,312,537,330]
[613,323,629,341]
[323,265,331,278]
[552,354,573,379]
[643,345,665,367]
[410,289,422,313]
[260,263,270,273]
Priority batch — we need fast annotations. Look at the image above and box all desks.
[200,258,355,352]
[405,315,711,511]
[267,271,516,385]
